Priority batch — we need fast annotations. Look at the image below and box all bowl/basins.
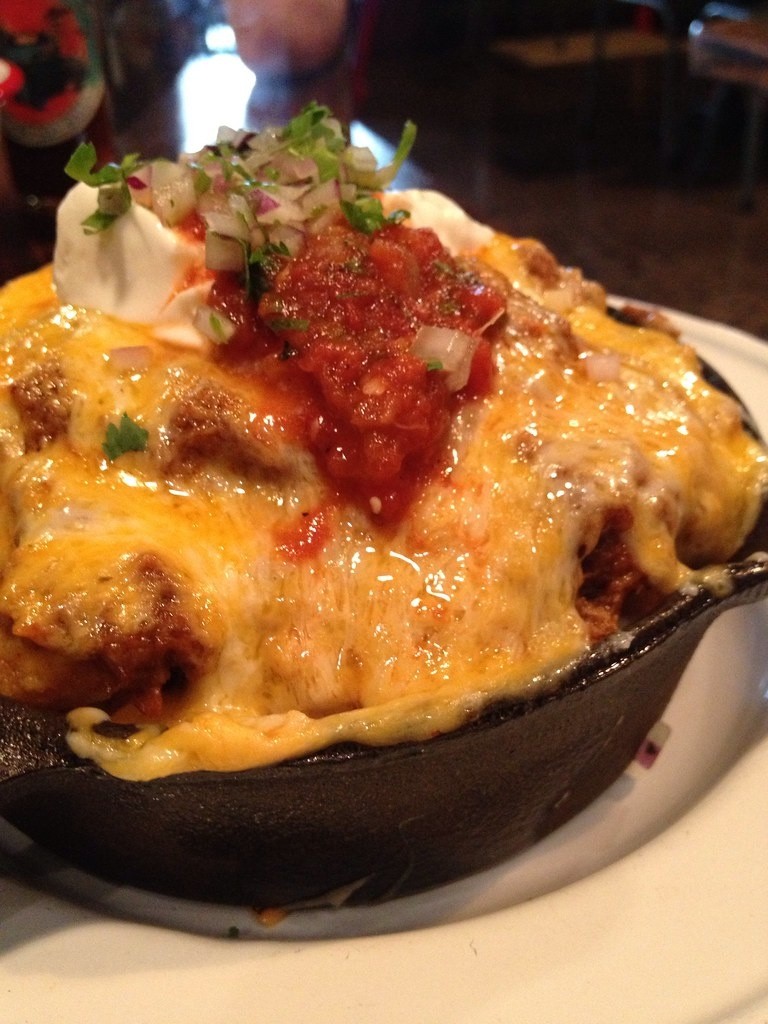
[0,311,768,911]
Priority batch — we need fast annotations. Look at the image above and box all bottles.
[0,1,123,243]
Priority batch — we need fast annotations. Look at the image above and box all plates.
[3,297,768,1024]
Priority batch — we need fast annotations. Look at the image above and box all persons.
[223,0,768,83]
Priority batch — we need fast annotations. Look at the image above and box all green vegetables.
[67,98,417,454]
